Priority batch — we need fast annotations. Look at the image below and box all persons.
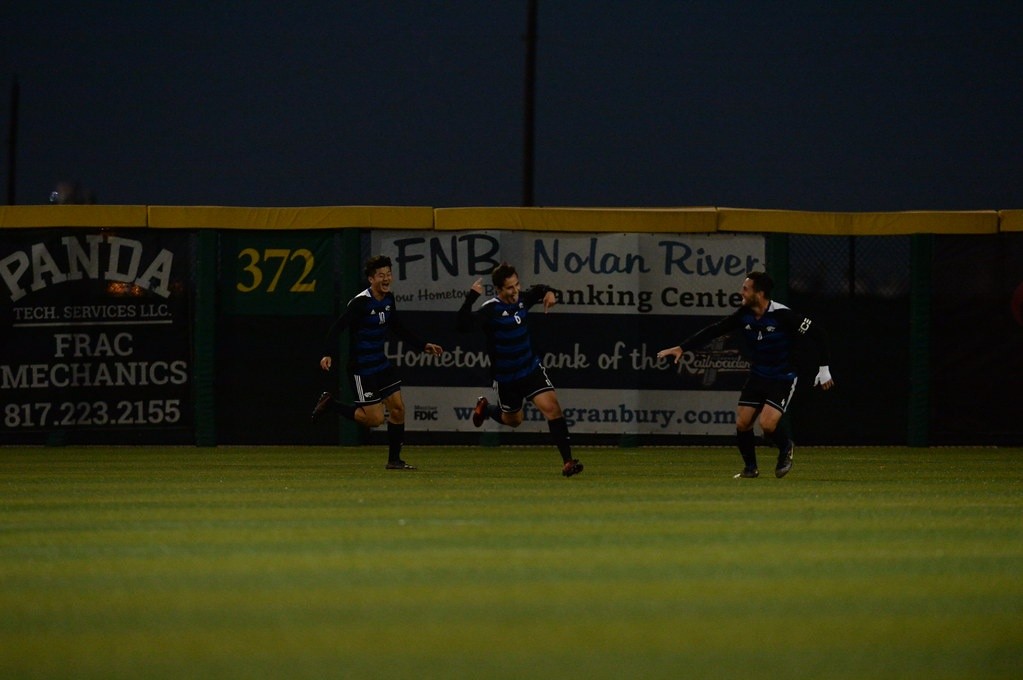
[657,271,834,478]
[310,255,443,471]
[448,263,584,477]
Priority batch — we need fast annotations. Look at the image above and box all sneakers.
[312,392,334,422]
[386,461,417,471]
[733,468,759,478]
[775,439,794,477]
[562,459,584,477]
[473,397,488,427]
